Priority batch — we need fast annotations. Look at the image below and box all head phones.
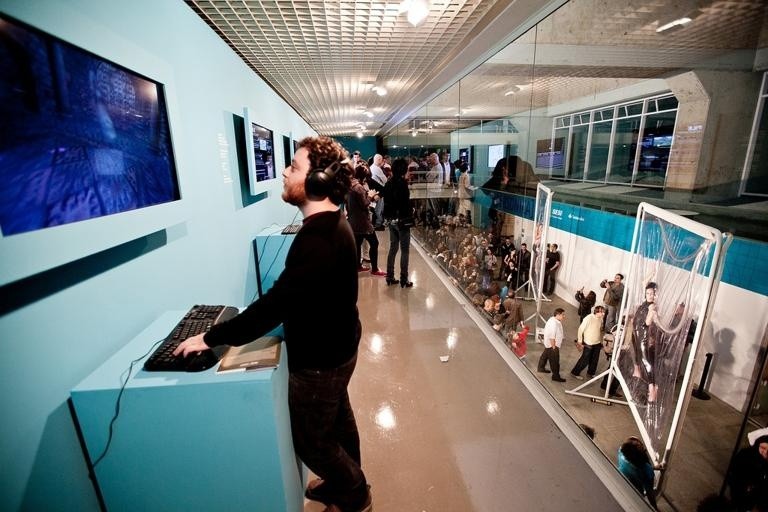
[304,156,340,199]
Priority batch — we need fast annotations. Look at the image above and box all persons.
[431,209,566,365]
[570,306,606,380]
[631,281,660,402]
[574,287,596,346]
[537,308,566,381]
[169,138,374,511]
[601,316,634,397]
[351,146,474,288]
[726,434,768,512]
[663,302,699,380]
[600,273,624,331]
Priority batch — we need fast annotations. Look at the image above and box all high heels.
[386,279,399,285]
[401,280,413,288]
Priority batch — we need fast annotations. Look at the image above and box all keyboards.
[144,304,238,372]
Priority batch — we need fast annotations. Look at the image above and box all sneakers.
[372,271,384,275]
[571,373,584,380]
[358,265,370,271]
[586,374,596,377]
[307,479,328,500]
[322,483,373,511]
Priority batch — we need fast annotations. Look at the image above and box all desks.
[255,223,301,294]
[68,308,309,511]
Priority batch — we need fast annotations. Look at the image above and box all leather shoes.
[538,368,551,373]
[609,392,623,398]
[552,374,566,382]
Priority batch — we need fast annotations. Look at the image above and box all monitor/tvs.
[457,148,471,172]
[534,136,566,170]
[243,106,278,197]
[0,12,187,292]
[487,145,504,168]
[288,131,300,162]
[628,125,673,171]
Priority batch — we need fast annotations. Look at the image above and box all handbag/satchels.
[492,263,498,270]
[398,217,415,232]
